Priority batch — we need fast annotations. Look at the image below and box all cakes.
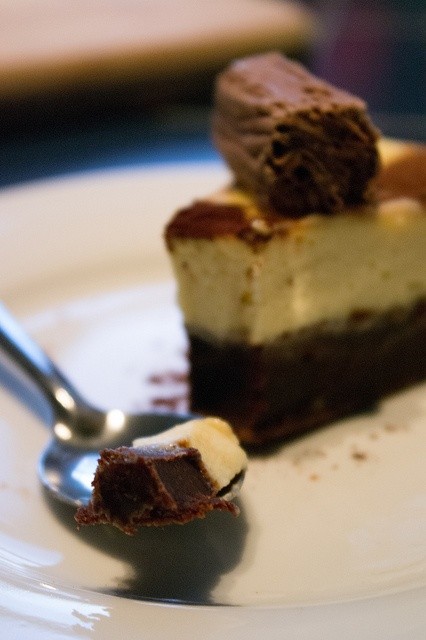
[165,142,425,451]
[208,52,378,219]
[72,416,245,537]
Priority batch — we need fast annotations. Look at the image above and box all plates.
[0,162,425,640]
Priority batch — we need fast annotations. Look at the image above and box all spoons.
[0,307,246,509]
